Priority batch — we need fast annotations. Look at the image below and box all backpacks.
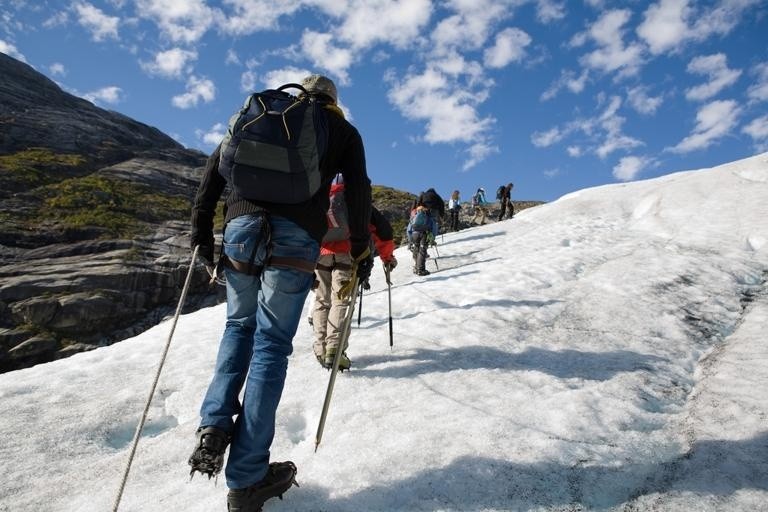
[322,191,353,244]
[471,194,478,206]
[496,185,505,199]
[216,81,329,211]
[411,213,428,231]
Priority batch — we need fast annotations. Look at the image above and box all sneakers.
[227,461,297,512]
[313,344,331,371]
[325,348,351,370]
[188,426,230,477]
[413,268,430,276]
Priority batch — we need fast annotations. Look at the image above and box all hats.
[297,75,338,101]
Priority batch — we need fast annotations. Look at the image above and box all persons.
[418,188,443,230]
[447,191,461,231]
[406,203,442,275]
[467,187,491,225]
[496,182,514,222]
[184,75,377,511]
[309,171,398,370]
